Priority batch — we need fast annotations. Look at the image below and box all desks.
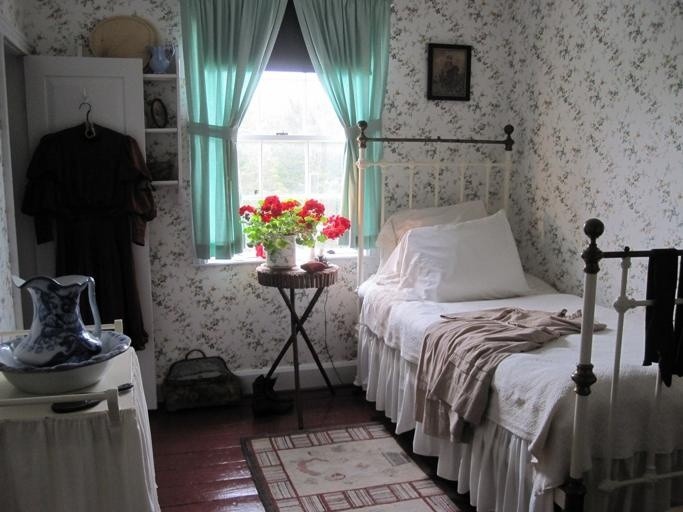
[247,261,344,432]
[0,330,166,512]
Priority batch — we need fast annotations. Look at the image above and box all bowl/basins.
[0,327,132,393]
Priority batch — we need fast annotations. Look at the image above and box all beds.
[351,113,681,512]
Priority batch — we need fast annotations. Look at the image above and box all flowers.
[237,190,351,260]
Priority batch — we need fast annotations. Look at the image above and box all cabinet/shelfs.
[143,72,182,185]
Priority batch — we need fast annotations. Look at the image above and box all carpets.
[235,417,466,512]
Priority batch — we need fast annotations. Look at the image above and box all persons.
[444,54,454,70]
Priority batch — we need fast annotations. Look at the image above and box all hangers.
[66,94,109,142]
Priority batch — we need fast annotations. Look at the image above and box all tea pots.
[10,270,104,361]
[146,44,175,75]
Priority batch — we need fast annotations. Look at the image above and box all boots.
[251,374,295,420]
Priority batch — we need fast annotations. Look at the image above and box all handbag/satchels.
[160,348,242,414]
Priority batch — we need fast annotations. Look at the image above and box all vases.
[261,232,298,271]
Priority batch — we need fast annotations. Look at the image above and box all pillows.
[391,204,535,304]
[378,194,487,251]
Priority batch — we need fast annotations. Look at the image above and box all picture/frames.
[421,41,477,105]
